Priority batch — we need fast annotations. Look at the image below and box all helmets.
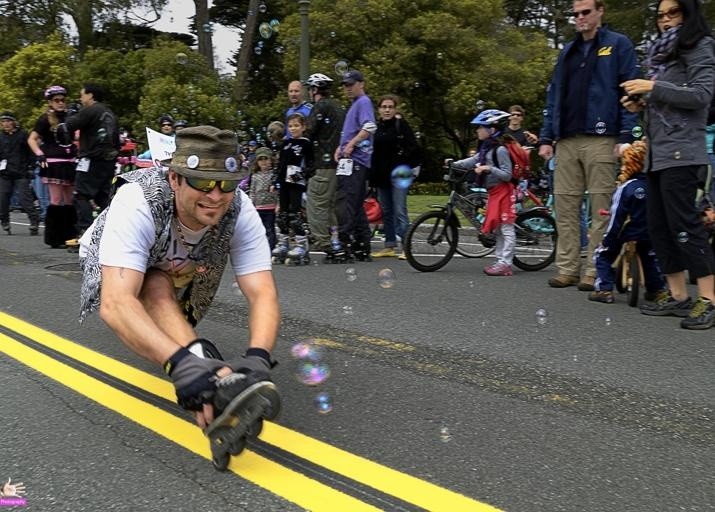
[44,86,68,98]
[174,119,189,128]
[303,72,334,88]
[469,109,512,129]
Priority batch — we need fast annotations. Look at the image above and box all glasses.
[512,114,522,117]
[54,98,65,103]
[570,9,590,17]
[184,178,242,194]
[655,6,680,22]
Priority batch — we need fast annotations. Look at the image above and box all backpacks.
[492,141,532,185]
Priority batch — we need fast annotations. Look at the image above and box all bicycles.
[401,161,594,273]
[599,209,641,309]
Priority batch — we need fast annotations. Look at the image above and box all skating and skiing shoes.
[271,234,371,266]
[2,222,11,234]
[29,221,39,235]
[184,339,283,471]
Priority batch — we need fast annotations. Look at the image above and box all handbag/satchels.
[396,120,432,182]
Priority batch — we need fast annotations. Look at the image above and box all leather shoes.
[398,254,407,260]
[368,250,394,257]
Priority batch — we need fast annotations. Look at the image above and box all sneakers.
[484,266,496,273]
[486,264,512,276]
[550,272,715,332]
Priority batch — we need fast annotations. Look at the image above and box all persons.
[77,122,284,437]
[1,3,715,332]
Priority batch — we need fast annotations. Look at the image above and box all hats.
[0,112,16,121]
[255,146,273,160]
[248,140,257,147]
[339,70,363,86]
[390,165,414,189]
[158,125,251,182]
